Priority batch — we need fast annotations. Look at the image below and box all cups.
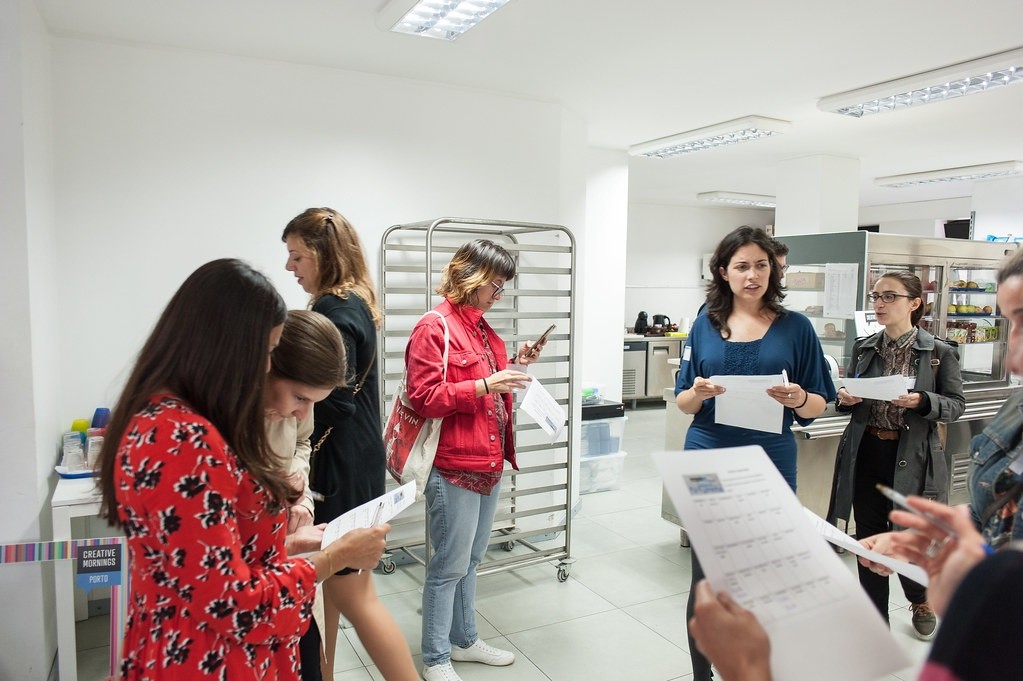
[63,408,110,473]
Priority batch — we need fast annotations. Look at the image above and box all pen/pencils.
[876,483,998,554]
[782,368,792,399]
[357,502,384,573]
[837,373,861,407]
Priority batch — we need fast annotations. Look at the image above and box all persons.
[673,226,837,681]
[847,246,1023,577]
[281,207,422,680]
[826,270,964,641]
[256,309,347,680]
[92,256,392,680]
[687,494,1023,681]
[404,238,550,680]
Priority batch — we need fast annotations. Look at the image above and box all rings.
[924,539,939,559]
[939,533,952,548]
[788,393,792,399]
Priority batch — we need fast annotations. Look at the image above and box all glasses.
[866,292,913,303]
[781,265,789,273]
[491,281,504,298]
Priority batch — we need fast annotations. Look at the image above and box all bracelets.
[482,378,489,395]
[321,548,332,577]
[795,389,809,409]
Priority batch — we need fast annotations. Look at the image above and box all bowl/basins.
[648,328,662,334]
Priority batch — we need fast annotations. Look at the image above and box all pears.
[949,304,992,314]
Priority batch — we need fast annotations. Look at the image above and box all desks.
[51,478,116,681]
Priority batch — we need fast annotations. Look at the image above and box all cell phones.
[524,325,556,357]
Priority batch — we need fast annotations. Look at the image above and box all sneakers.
[450,638,514,665]
[422,662,462,681]
[909,601,938,640]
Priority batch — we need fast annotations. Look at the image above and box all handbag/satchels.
[383,310,450,495]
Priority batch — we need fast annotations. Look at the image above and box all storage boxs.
[578,399,653,489]
[786,272,824,288]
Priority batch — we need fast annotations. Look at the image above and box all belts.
[865,427,898,441]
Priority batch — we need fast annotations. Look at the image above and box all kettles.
[653,315,670,328]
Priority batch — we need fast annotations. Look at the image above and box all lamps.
[873,160,1023,189]
[697,190,777,208]
[376,1,520,49]
[818,48,1022,119]
[626,115,790,160]
